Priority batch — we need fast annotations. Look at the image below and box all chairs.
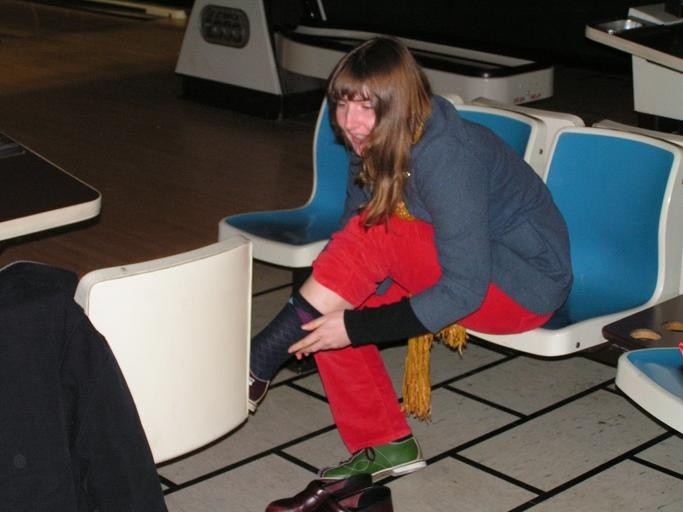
[212,96,683,439]
[78,231,259,466]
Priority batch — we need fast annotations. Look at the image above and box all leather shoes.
[319,435,427,487]
[249,369,271,412]
[265,474,393,512]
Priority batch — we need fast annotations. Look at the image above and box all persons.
[249,33,575,483]
